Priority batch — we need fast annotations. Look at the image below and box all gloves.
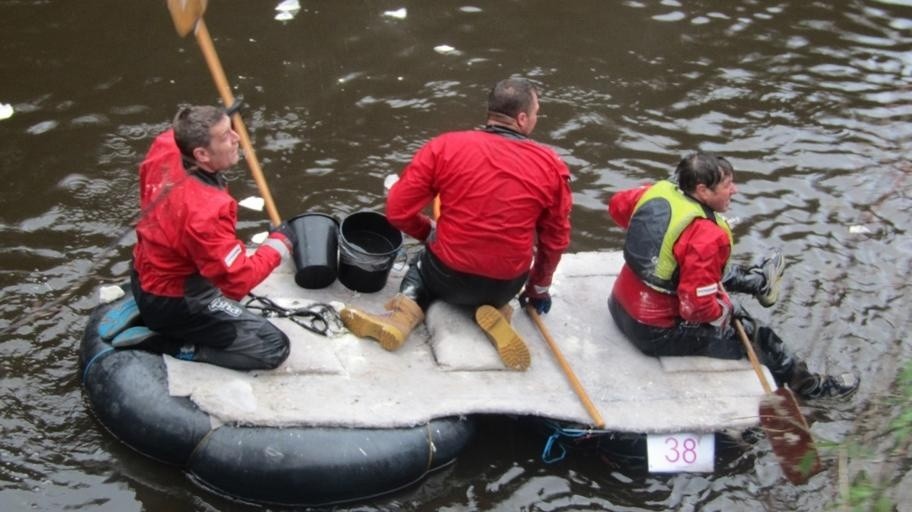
[518,291,551,315]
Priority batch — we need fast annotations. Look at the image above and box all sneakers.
[112,326,177,356]
[96,297,147,342]
[748,248,785,307]
[794,370,860,403]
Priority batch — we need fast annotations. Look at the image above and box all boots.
[475,303,532,372]
[340,292,425,351]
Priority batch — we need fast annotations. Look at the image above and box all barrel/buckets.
[286,212,341,289]
[336,207,404,294]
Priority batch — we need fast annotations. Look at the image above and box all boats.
[78,262,783,509]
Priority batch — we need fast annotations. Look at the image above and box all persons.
[608,151,854,400]
[338,78,573,373]
[95,103,299,374]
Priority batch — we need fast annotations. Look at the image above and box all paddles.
[719,280,822,485]
[167,0,281,228]
